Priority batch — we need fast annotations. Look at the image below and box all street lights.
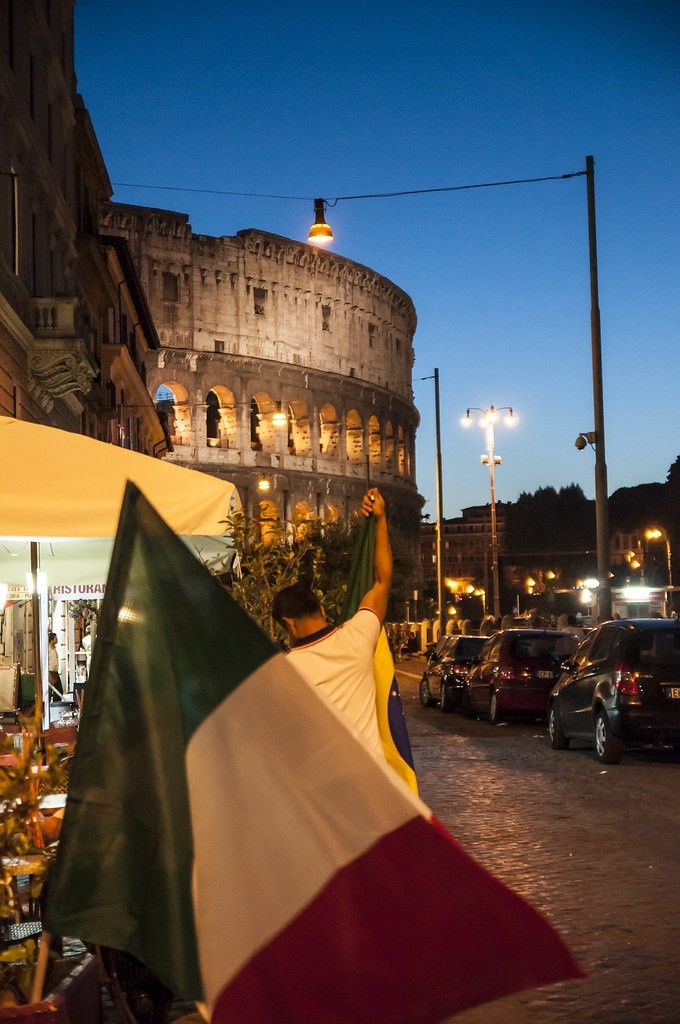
[460,404,518,621]
[647,528,673,604]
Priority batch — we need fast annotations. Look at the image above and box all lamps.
[306,199,333,244]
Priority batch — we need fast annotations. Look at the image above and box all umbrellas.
[1,416,246,601]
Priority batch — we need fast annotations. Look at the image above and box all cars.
[462,629,580,724]
[420,635,491,712]
[546,617,680,764]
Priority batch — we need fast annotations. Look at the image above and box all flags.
[41,478,587,1024]
[340,510,419,798]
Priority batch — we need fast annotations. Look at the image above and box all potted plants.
[0,694,102,1024]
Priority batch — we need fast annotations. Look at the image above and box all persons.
[400,631,418,660]
[556,612,663,643]
[47,632,63,703]
[82,624,91,680]
[271,485,395,772]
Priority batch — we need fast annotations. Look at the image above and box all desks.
[1,725,81,878]
